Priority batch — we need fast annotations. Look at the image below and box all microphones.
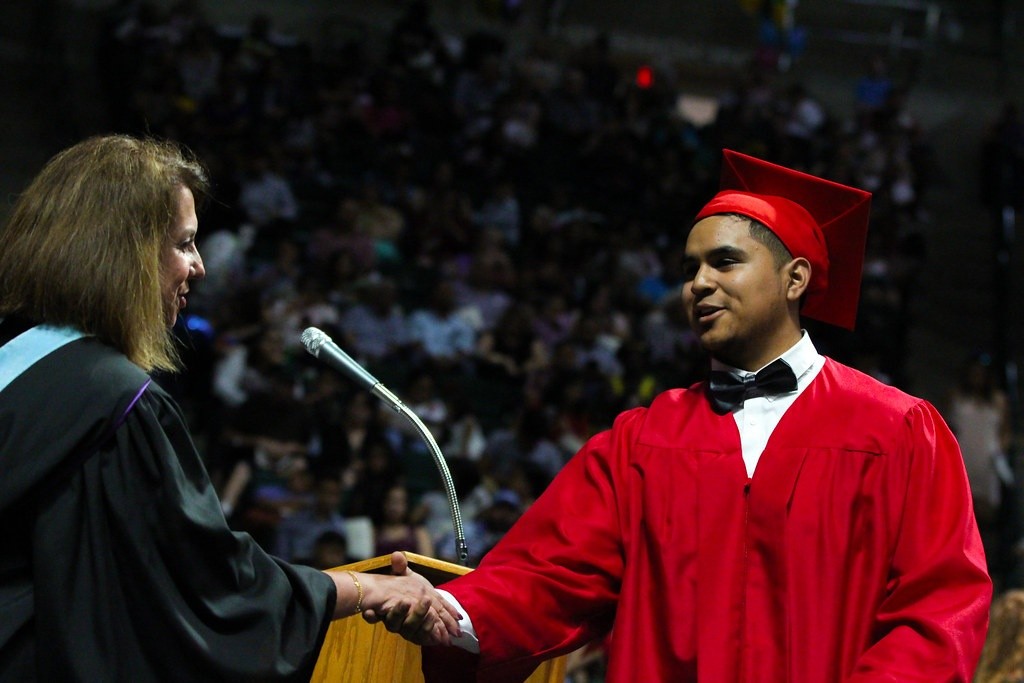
[299,327,468,569]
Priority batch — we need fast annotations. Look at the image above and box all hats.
[692,148,871,332]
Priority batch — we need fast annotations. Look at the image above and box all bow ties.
[700,358,797,416]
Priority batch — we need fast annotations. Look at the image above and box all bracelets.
[344,569,361,614]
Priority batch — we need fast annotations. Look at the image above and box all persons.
[0,136,463,683]
[363,146,994,683]
[0,0,1024,683]
